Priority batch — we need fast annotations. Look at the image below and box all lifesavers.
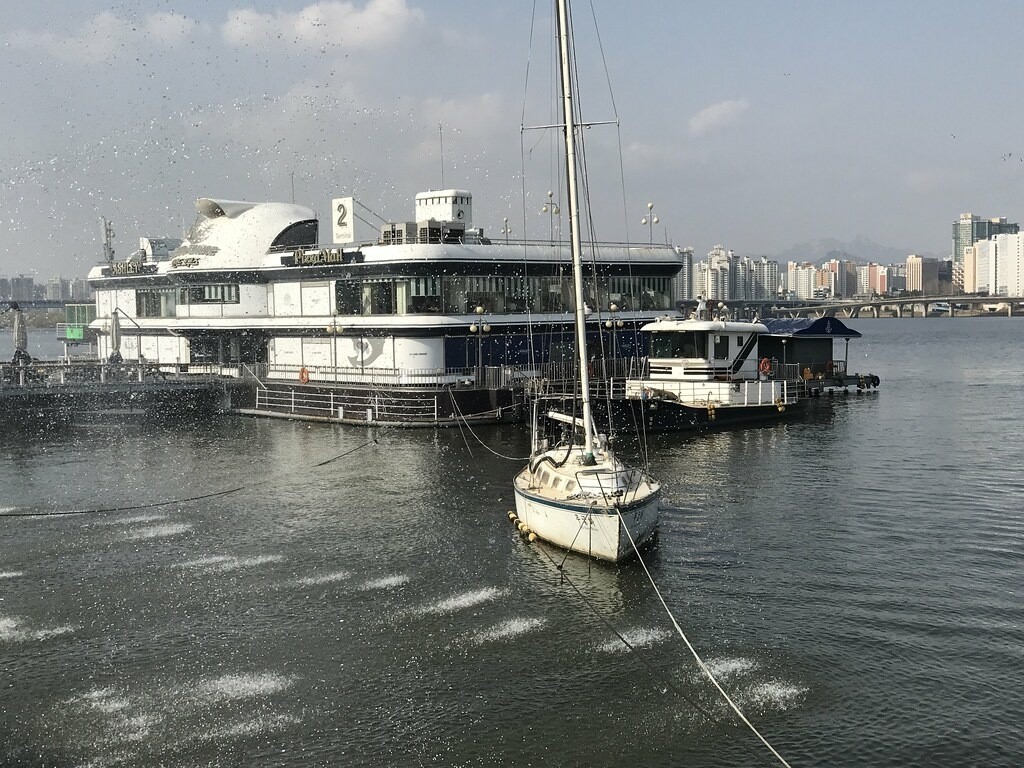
[299,368,308,383]
[760,358,771,375]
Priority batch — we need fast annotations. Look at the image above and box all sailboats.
[512,0,661,566]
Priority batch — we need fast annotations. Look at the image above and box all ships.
[86,188,800,429]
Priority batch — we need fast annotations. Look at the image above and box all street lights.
[542,190,560,240]
[603,303,623,378]
[325,310,344,387]
[501,217,513,246]
[642,202,659,243]
[470,306,490,387]
